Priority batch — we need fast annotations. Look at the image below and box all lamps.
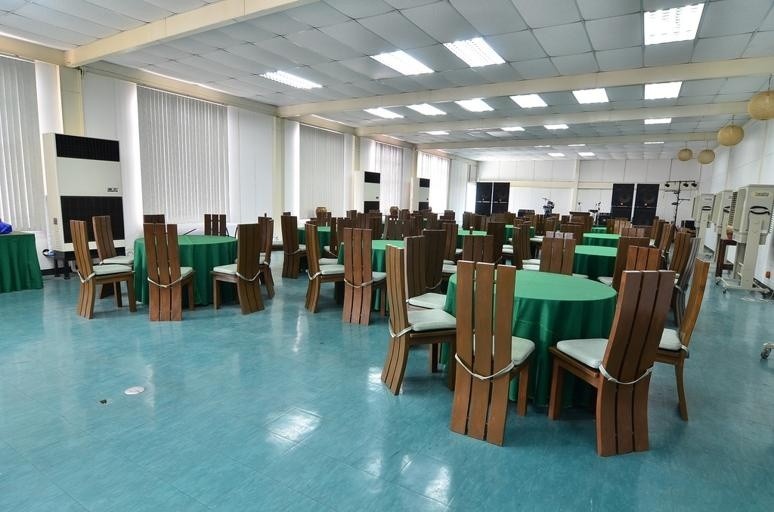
[698,141,715,164]
[716,114,744,145]
[748,74,773,123]
[679,142,692,162]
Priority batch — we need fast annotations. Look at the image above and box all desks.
[0,230,44,292]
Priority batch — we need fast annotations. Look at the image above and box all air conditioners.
[352,168,381,212]
[45,134,125,251]
[411,177,430,211]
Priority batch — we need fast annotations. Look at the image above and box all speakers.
[633,183,660,226]
[611,183,635,220]
[474,181,492,215]
[492,182,510,213]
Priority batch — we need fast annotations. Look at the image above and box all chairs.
[67,210,275,323]
[283,207,711,455]
